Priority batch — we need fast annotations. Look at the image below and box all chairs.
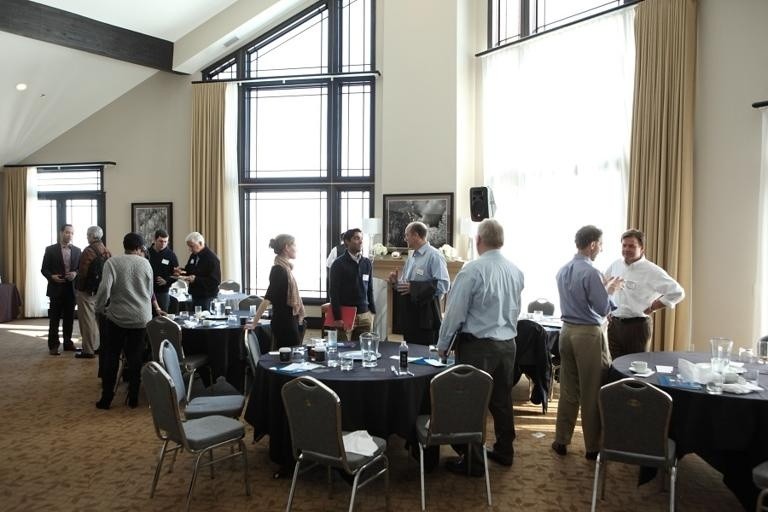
[415,365,495,511]
[281,376,391,512]
[139,282,309,512]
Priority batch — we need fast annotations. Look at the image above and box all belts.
[615,317,649,322]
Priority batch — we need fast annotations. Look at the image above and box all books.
[655,374,703,391]
[445,332,458,357]
[268,360,326,375]
[323,304,358,331]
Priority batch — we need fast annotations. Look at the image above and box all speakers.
[470,187,496,221]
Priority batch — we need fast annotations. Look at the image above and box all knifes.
[391,365,398,376]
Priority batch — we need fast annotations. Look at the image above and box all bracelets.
[648,306,656,312]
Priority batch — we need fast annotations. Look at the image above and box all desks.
[371,256,465,341]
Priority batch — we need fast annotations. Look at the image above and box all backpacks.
[85,245,111,296]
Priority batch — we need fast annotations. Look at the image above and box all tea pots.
[210,298,227,315]
[359,331,380,367]
[709,337,733,371]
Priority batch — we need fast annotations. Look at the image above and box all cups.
[340,354,354,371]
[534,310,544,321]
[739,341,768,386]
[631,361,647,371]
[307,338,327,363]
[279,347,293,363]
[179,296,256,326]
[325,344,338,367]
[292,347,305,362]
[706,375,724,395]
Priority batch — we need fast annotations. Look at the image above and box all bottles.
[399,340,408,376]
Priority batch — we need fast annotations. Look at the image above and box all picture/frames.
[131,202,173,250]
[382,192,455,254]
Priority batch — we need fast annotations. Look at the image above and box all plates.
[628,367,651,373]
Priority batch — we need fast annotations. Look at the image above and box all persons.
[550,225,624,459]
[599,228,685,362]
[382,222,451,345]
[243,233,307,354]
[38,222,222,412]
[330,228,378,343]
[435,219,523,473]
[325,233,346,267]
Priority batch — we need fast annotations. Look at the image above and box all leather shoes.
[586,452,598,460]
[445,456,485,476]
[50,345,100,359]
[552,441,566,455]
[128,392,138,409]
[96,392,113,409]
[487,447,512,465]
